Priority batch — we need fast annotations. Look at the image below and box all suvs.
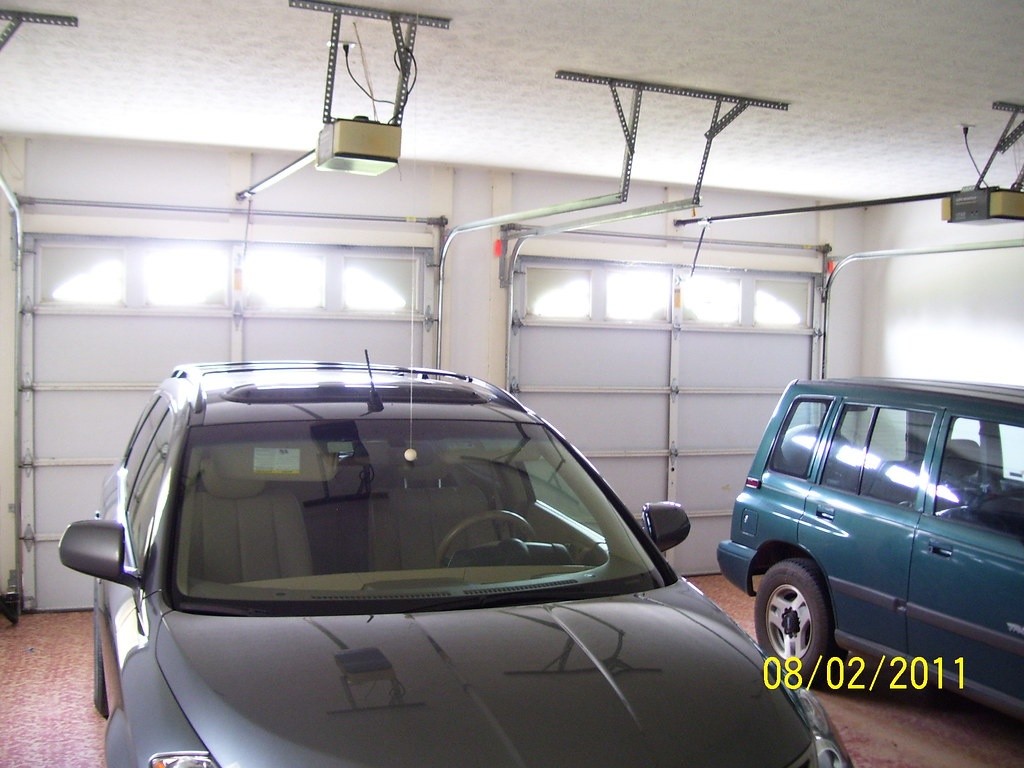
[57,361,854,768]
[717,376,1024,722]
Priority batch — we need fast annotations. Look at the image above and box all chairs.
[366,434,515,571]
[184,458,312,594]
[941,438,985,508]
